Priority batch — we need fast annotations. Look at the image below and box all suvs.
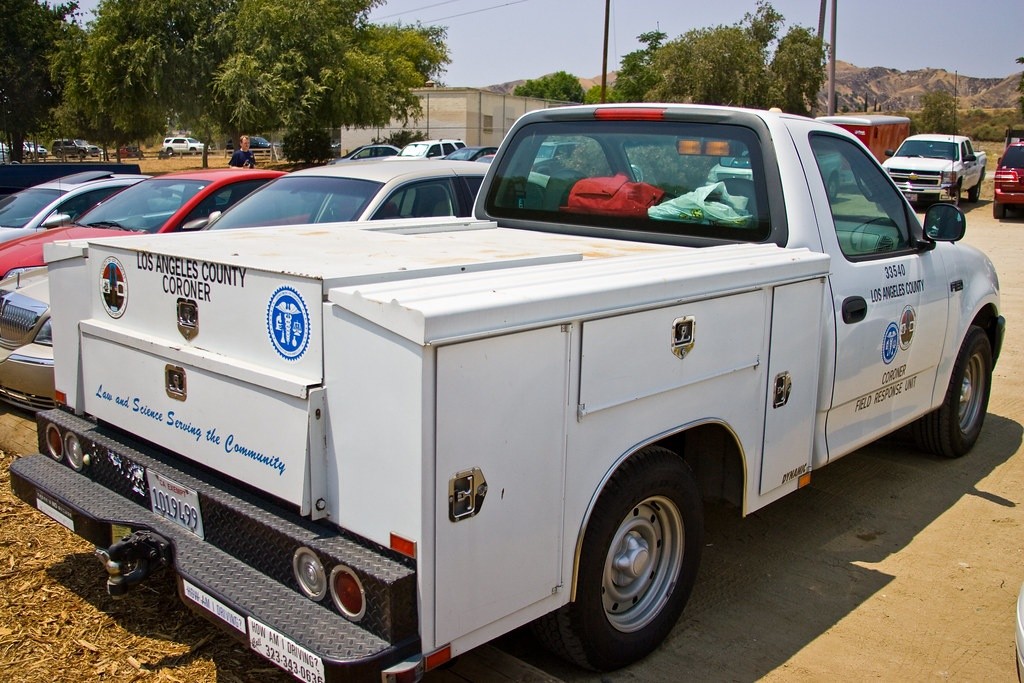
[51,139,101,159]
[391,138,467,159]
[161,137,211,156]
[226,136,272,154]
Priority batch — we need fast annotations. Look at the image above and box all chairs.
[173,186,217,232]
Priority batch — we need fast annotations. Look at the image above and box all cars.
[91,149,109,157]
[702,146,842,205]
[119,146,144,158]
[0,141,48,162]
[324,143,396,160]
[993,142,1024,219]
[441,138,579,173]
[0,157,550,425]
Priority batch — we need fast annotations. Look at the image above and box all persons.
[228,135,256,170]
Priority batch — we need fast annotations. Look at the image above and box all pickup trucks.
[9,101,1005,682]
[880,133,987,205]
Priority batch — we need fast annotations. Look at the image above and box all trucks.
[811,114,911,203]
